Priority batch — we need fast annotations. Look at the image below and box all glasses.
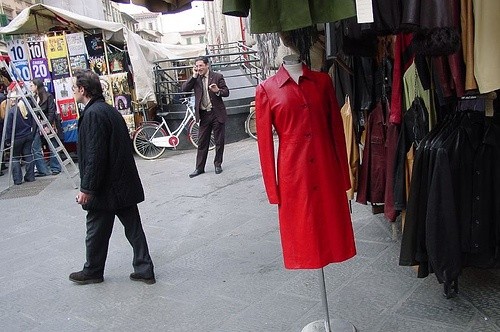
[30,85,35,86]
[72,85,80,90]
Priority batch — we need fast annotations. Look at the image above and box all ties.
[201,76,210,108]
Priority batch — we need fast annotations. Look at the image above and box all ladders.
[0,60,81,190]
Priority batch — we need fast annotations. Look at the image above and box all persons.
[0,78,64,186]
[181,56,229,177]
[68,68,156,285]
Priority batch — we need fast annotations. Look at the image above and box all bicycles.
[132,97,216,160]
[246,109,276,141]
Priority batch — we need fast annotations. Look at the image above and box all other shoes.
[35,172,45,176]
[1,166,8,169]
[24,178,34,182]
[190,169,205,176]
[52,171,60,174]
[70,272,105,284]
[14,182,22,185]
[0,173,4,176]
[215,166,222,174]
[130,273,155,285]
